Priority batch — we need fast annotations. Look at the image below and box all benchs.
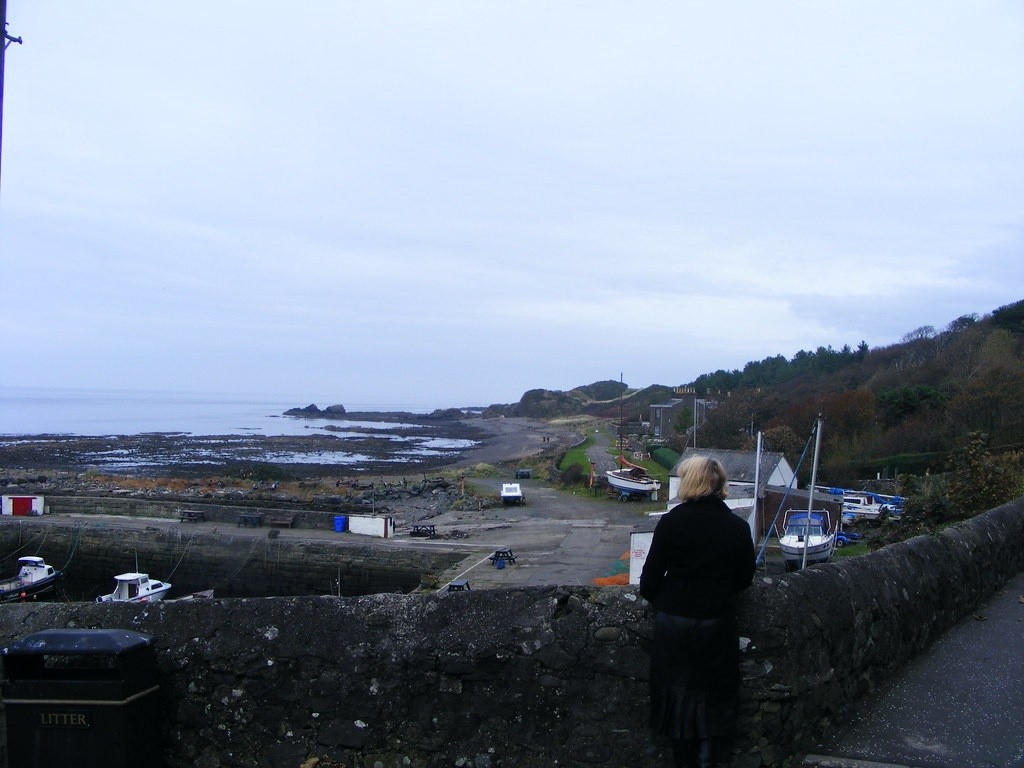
[489,554,496,560]
[178,515,206,520]
[511,553,519,561]
[270,517,293,528]
[410,528,438,535]
[237,520,263,525]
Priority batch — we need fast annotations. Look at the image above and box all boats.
[778,508,837,568]
[606,467,662,490]
[96,546,174,602]
[0,555,64,602]
[838,495,905,523]
[500,482,525,505]
[175,588,215,600]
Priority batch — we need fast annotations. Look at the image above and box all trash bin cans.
[0,629,166,768]
[334,516,345,532]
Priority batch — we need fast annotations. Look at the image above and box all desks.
[238,515,262,528]
[447,580,471,592]
[492,548,516,566]
[411,524,436,539]
[180,511,205,523]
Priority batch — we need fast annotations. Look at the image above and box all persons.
[640,454,758,768]
